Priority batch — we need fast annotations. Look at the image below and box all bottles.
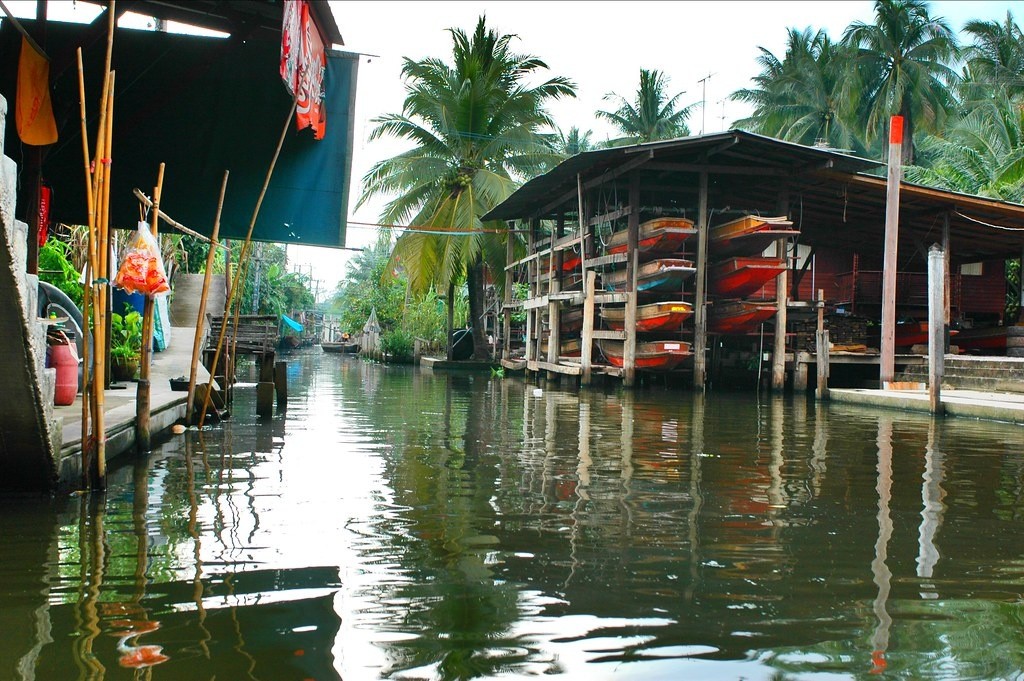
[50,312,57,319]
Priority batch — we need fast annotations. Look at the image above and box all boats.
[524,214,796,371]
[320,339,361,354]
[764,339,867,354]
[868,325,959,348]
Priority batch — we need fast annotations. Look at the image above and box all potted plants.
[111,310,144,382]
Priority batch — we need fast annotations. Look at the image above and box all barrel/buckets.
[50,331,79,406]
